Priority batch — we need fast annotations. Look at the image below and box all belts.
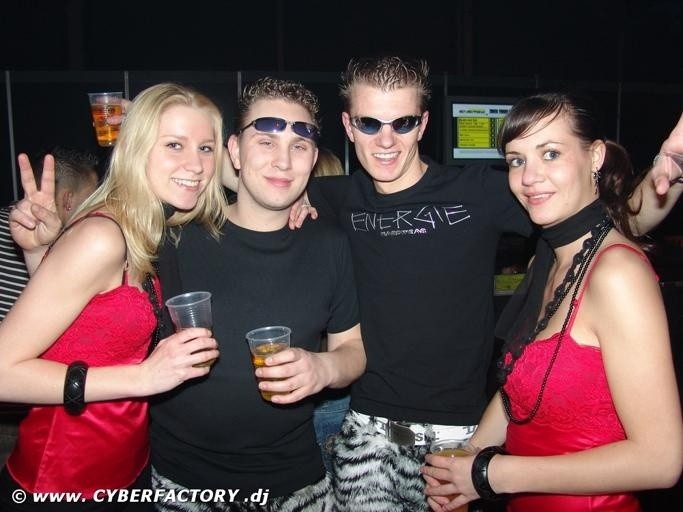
[347,405,479,446]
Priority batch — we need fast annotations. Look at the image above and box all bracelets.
[64,361,87,417]
[470,445,505,500]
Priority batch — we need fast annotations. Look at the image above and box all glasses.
[349,115,423,135]
[237,115,323,145]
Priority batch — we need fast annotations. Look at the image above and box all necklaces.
[140,257,164,349]
[496,225,613,425]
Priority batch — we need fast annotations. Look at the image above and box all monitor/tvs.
[442,95,520,165]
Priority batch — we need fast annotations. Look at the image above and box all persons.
[94,53,683,512]
[1,83,223,511]
[303,147,352,474]
[9,73,368,511]
[423,91,681,511]
[0,144,98,320]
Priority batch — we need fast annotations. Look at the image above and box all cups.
[427,439,476,511]
[244,325,293,402]
[164,289,211,365]
[90,92,122,147]
